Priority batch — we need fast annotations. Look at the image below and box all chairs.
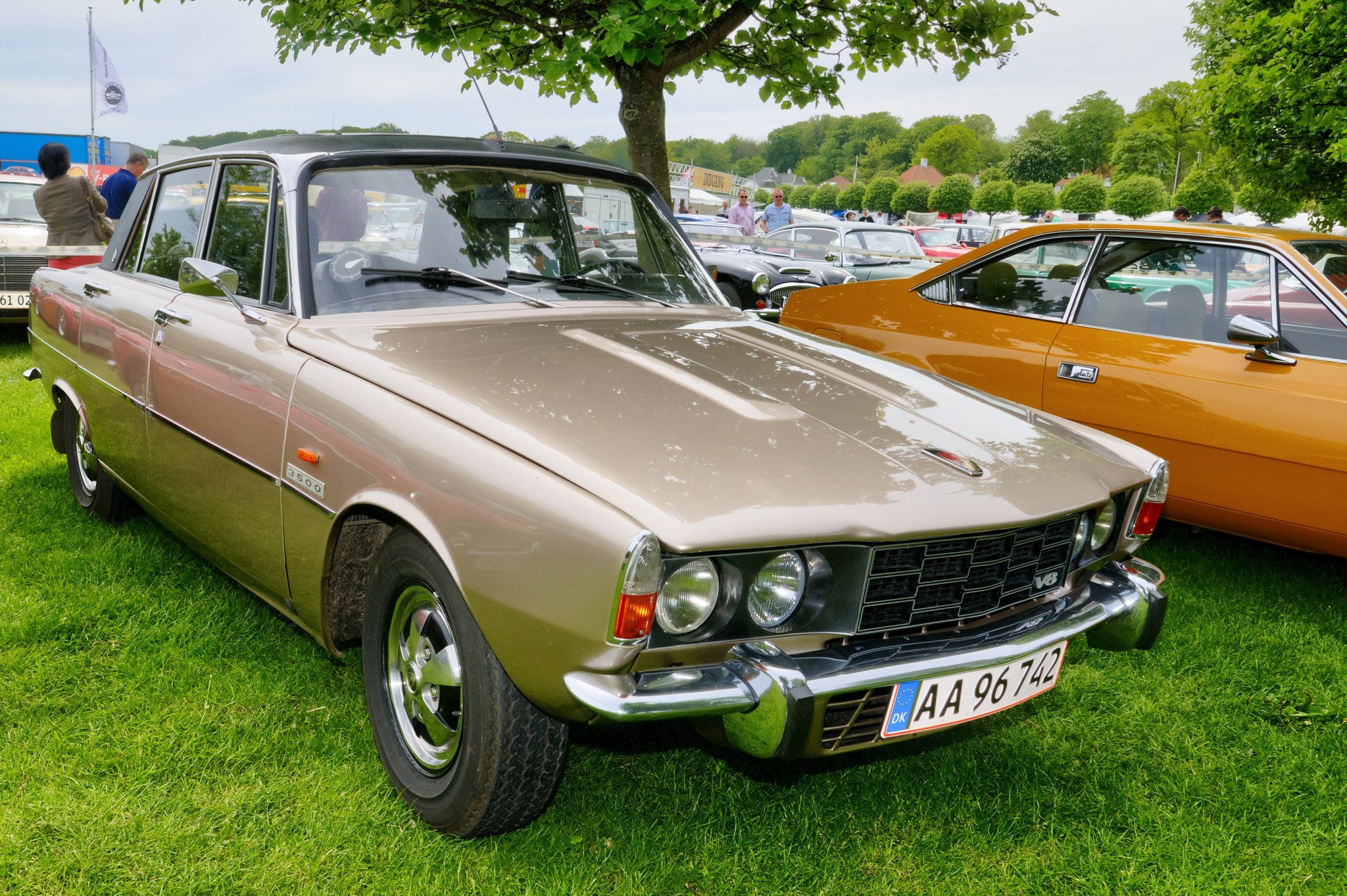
[1042,259,1092,315]
[970,260,1026,310]
[417,194,517,280]
[1156,287,1212,335]
[190,200,267,288]
[1096,288,1150,339]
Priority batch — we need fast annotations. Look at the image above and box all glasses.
[774,196,783,198]
[1046,213,1052,219]
[739,196,748,199]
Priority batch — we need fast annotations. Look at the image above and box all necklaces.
[1201,205,1235,273]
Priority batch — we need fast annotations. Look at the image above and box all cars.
[0,174,49,324]
[0,166,42,178]
[565,211,1273,323]
[774,221,1347,561]
[24,132,1169,839]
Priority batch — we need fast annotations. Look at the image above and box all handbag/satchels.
[78,175,116,242]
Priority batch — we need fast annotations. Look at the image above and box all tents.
[965,207,1347,238]
[688,188,724,214]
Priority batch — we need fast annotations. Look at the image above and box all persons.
[760,188,795,235]
[520,145,572,280]
[34,142,110,272]
[728,189,756,237]
[716,200,730,220]
[845,208,875,223]
[899,222,904,227]
[1150,205,1190,271]
[100,150,150,246]
[682,206,699,215]
[1044,208,1061,224]
[411,197,427,226]
[875,211,885,225]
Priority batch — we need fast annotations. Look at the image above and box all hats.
[863,208,868,213]
